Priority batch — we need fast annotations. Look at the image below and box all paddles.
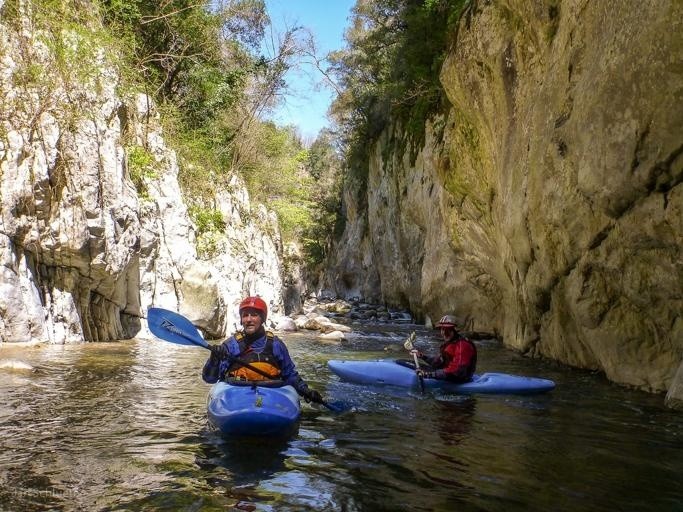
[403,330,425,395]
[147,308,352,412]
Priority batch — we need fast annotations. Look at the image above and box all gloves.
[308,388,322,404]
[409,349,422,358]
[210,344,227,366]
[416,370,430,378]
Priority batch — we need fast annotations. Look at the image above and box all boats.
[325,379,543,413]
[206,369,299,432]
[206,422,323,510]
[322,357,556,396]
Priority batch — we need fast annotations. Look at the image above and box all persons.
[203,297,323,406]
[411,315,478,385]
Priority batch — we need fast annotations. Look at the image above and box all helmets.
[435,315,461,332]
[238,296,267,321]
